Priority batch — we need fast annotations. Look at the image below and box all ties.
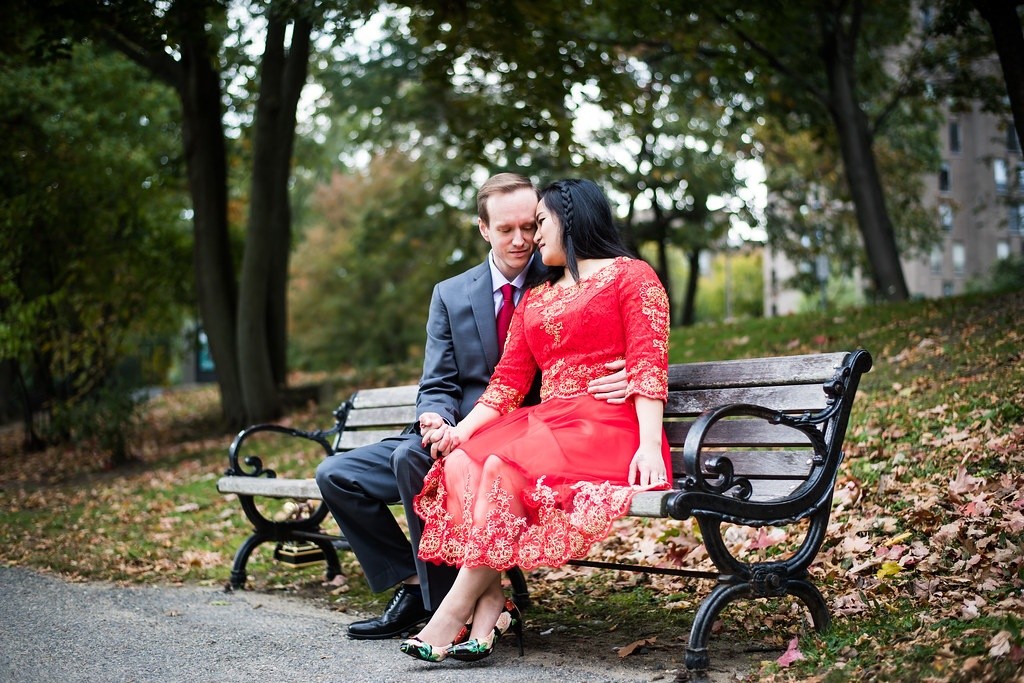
[497,284,515,361]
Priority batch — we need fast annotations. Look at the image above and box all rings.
[657,475,666,480]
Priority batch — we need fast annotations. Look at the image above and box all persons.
[400,177,674,663]
[313,171,632,641]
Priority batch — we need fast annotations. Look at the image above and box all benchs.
[216,352,876,672]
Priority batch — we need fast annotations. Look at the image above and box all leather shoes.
[348,584,433,639]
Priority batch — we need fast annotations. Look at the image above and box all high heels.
[444,600,526,662]
[400,610,474,664]
